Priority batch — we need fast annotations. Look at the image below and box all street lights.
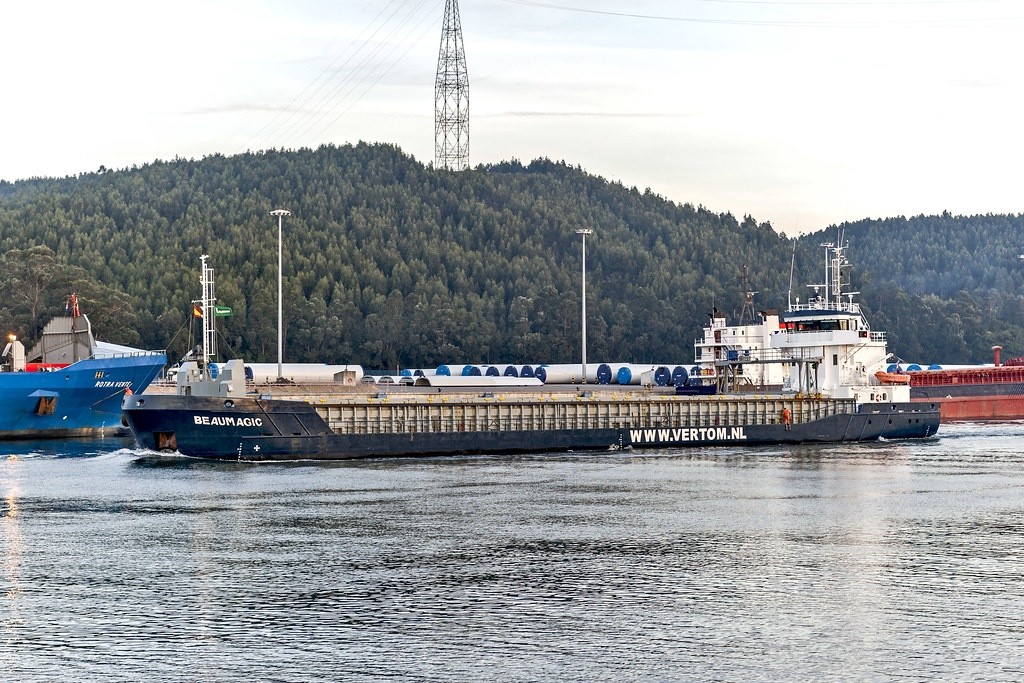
[268,208,291,380]
[574,227,594,383]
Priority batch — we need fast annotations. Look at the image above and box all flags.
[193,304,202,318]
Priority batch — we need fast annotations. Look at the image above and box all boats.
[0,340,167,436]
[768,229,1024,423]
[123,254,942,461]
[874,371,911,383]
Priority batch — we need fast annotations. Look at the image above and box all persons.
[783,408,791,424]
[125,387,133,395]
